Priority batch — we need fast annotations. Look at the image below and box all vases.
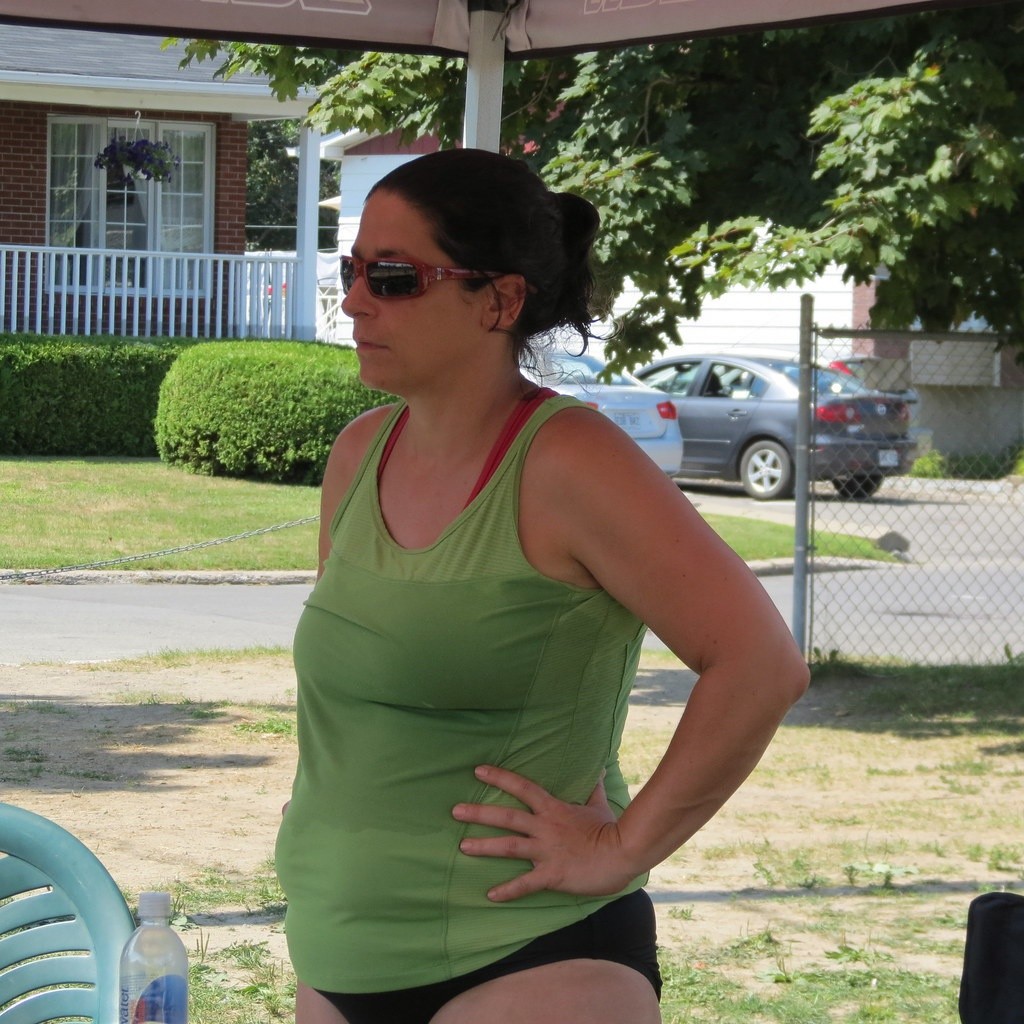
[123,163,154,180]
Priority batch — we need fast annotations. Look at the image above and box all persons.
[276,149,810,1024]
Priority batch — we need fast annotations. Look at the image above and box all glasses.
[339,255,538,301]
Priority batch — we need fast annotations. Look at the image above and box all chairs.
[706,371,728,397]
[0,802,137,1024]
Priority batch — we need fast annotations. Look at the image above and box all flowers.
[94,136,182,183]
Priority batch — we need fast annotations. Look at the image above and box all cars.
[513,348,688,486]
[623,352,920,501]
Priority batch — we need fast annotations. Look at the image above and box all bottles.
[118,892,188,1024]
